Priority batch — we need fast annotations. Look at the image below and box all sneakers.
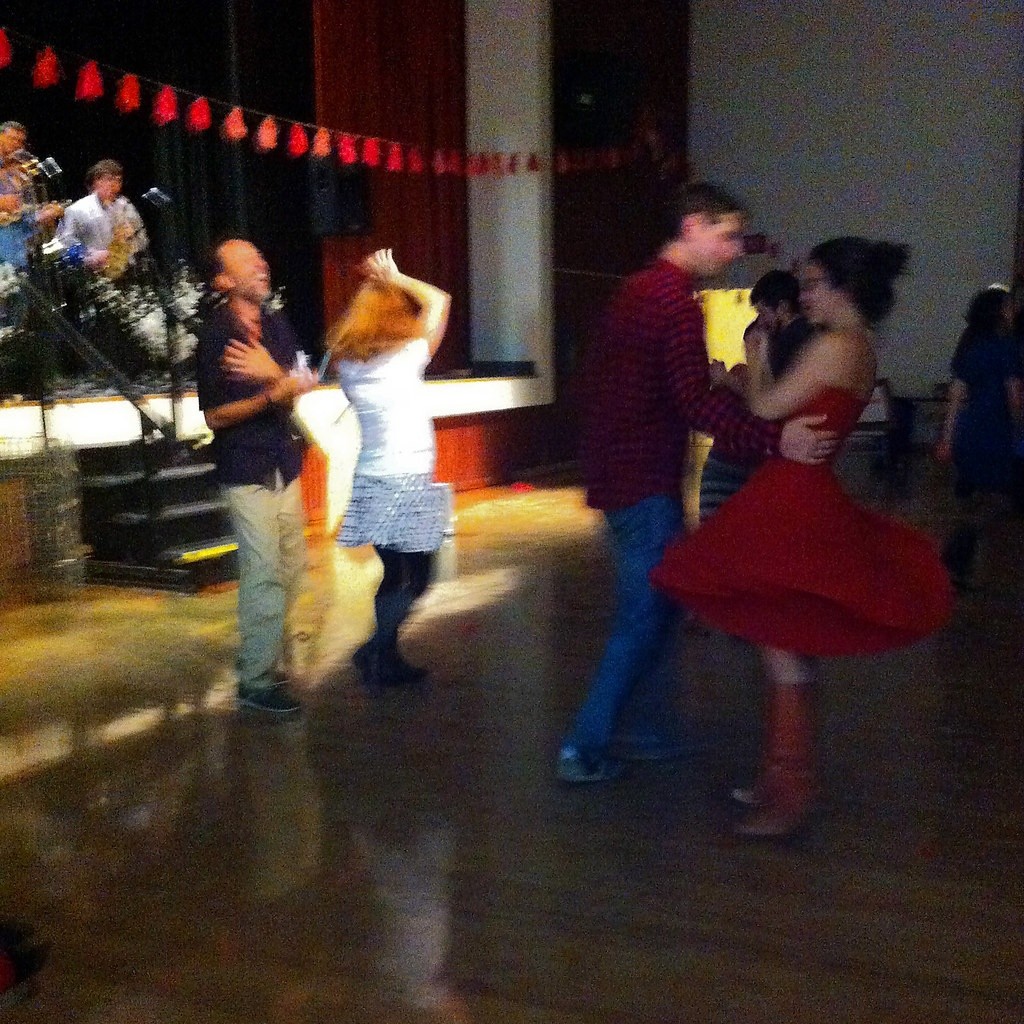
[233,660,292,685]
[236,682,300,714]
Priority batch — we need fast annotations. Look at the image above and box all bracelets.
[262,393,276,412]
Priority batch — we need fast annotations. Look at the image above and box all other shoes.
[726,771,829,839]
[552,736,634,784]
[616,723,689,762]
[375,647,429,683]
[940,523,983,586]
[351,642,385,700]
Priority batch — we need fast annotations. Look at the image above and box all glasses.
[797,274,831,290]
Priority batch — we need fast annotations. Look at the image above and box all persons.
[195,237,323,714]
[0,118,149,407]
[547,181,1024,840]
[327,245,453,695]
